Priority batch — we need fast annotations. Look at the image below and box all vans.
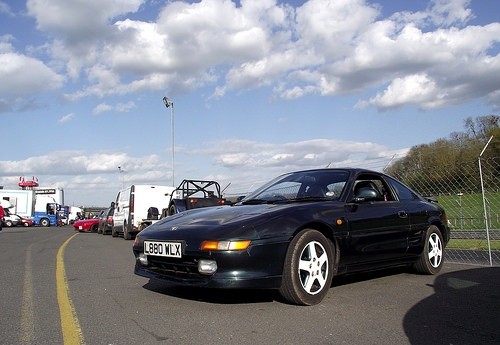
[112,185,176,240]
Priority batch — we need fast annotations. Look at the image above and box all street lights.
[163,95,175,187]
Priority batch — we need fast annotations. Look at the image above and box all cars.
[18,215,35,227]
[74,216,101,233]
[58,216,62,227]
[60,215,67,226]
[132,168,451,306]
[98,203,115,236]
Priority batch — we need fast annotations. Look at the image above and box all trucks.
[0,189,57,227]
[0,199,20,227]
[57,205,93,225]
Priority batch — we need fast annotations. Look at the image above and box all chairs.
[354,181,382,201]
[307,186,326,197]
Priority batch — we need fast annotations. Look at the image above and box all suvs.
[160,179,233,219]
